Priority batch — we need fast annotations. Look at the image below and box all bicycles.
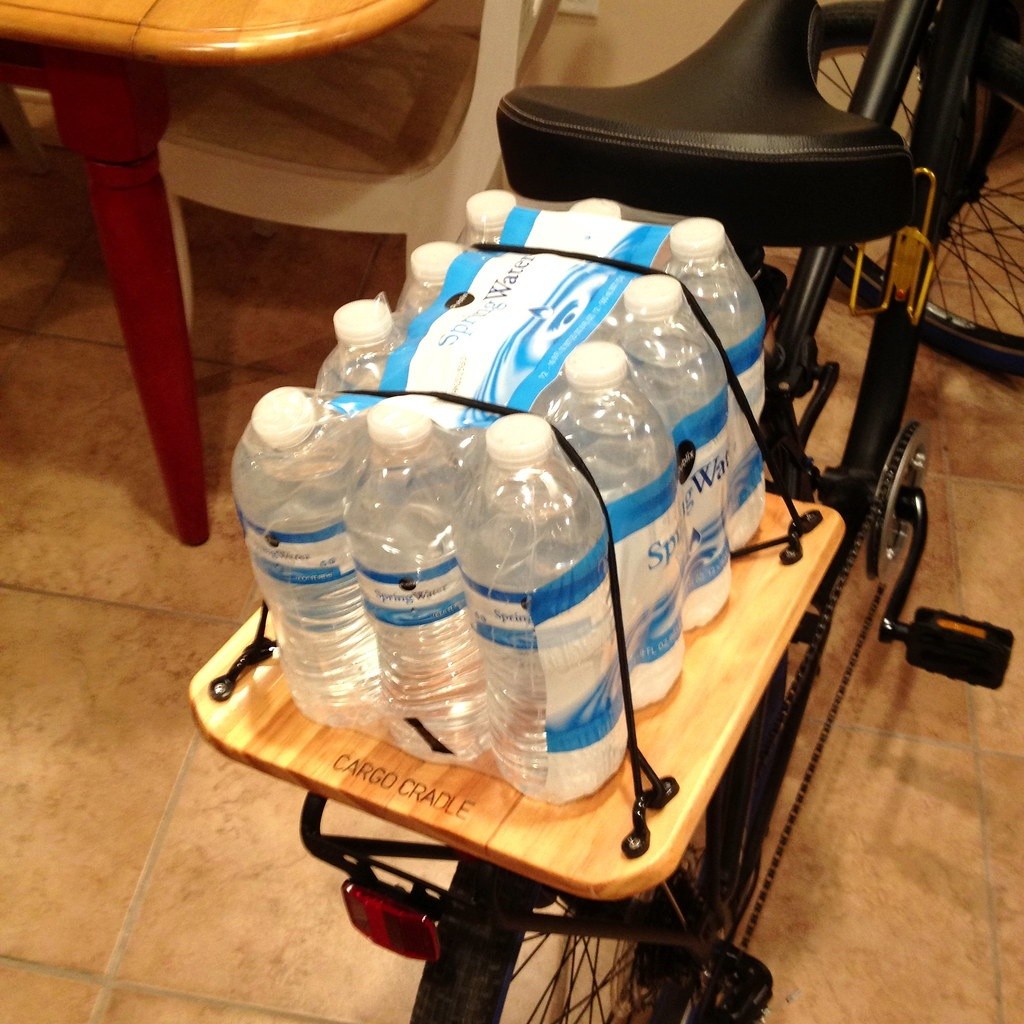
[185,2,1023,1023]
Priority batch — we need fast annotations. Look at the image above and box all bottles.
[229,189,768,803]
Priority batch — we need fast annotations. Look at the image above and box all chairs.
[0,1,554,346]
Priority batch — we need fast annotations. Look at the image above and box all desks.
[0,0,440,550]
